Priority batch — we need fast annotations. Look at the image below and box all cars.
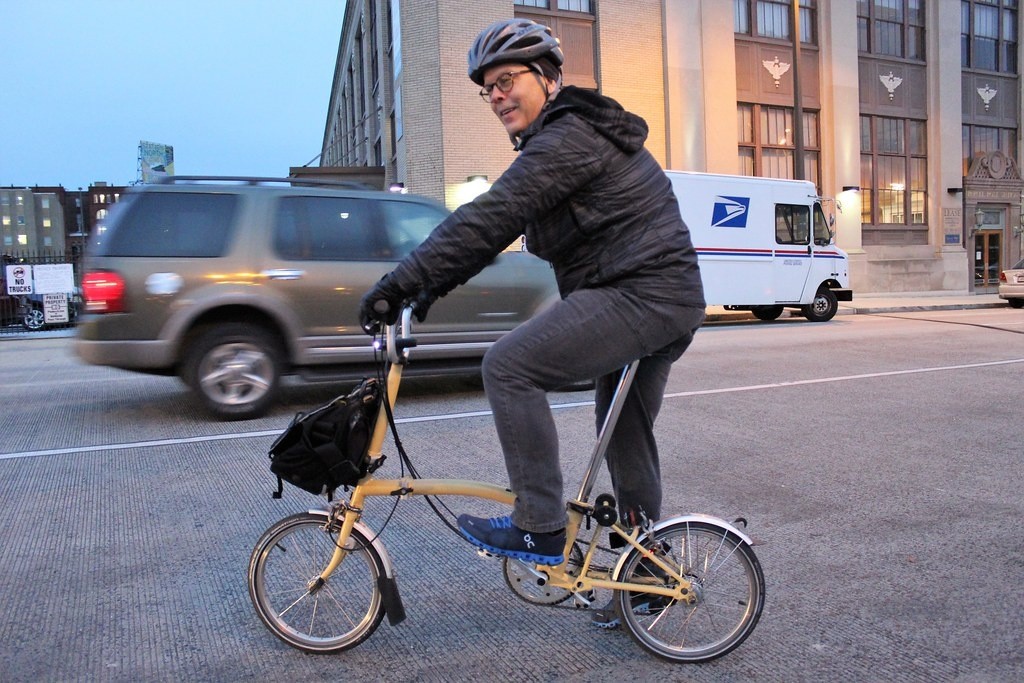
[0,261,82,331]
[998,256,1024,308]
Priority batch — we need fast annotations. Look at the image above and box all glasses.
[479,68,535,103]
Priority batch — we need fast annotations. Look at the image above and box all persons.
[360,17,705,626]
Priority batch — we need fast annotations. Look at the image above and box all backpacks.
[268,377,383,499]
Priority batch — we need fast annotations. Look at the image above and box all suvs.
[71,176,596,421]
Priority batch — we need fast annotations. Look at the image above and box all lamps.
[843,186,860,193]
[467,175,488,184]
[948,188,964,193]
[389,182,404,192]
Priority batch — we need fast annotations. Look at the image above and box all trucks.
[662,169,852,322]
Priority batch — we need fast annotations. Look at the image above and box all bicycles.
[247,292,765,663]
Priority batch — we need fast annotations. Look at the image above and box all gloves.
[359,272,405,336]
[413,288,439,323]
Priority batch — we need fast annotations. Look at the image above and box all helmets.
[467,18,564,86]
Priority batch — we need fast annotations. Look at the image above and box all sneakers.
[456,513,567,566]
[591,586,666,628]
